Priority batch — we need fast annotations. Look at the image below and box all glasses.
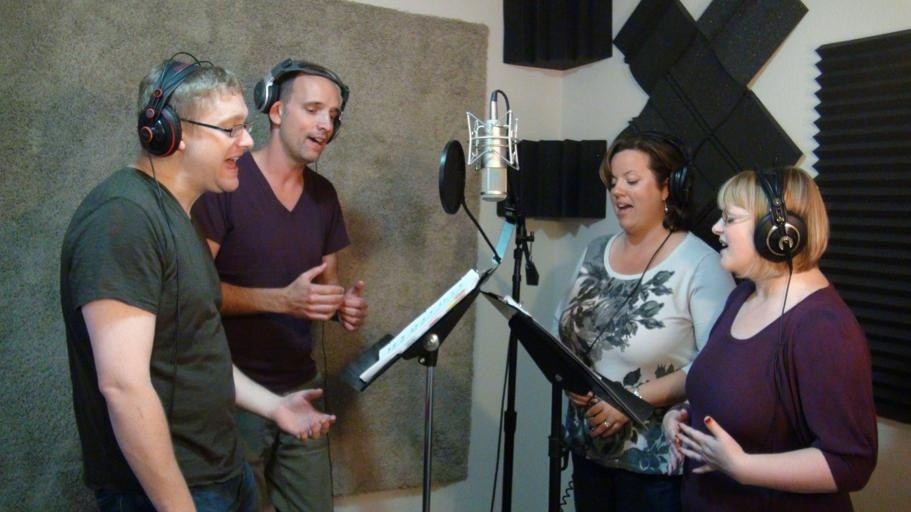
[178,118,256,137]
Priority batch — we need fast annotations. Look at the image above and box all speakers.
[503,0,612,71]
[497,139,607,218]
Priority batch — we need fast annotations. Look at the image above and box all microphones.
[466,107,521,203]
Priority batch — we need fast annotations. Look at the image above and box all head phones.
[138,52,216,158]
[755,160,808,263]
[254,58,350,144]
[643,130,696,206]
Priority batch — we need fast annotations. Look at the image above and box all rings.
[602,421,611,429]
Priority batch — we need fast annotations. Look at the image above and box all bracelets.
[629,385,643,401]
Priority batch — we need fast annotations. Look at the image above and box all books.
[357,264,481,383]
[495,287,650,435]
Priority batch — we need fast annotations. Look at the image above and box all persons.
[661,160,876,509]
[61,52,340,512]
[556,129,736,512]
[188,56,367,512]
